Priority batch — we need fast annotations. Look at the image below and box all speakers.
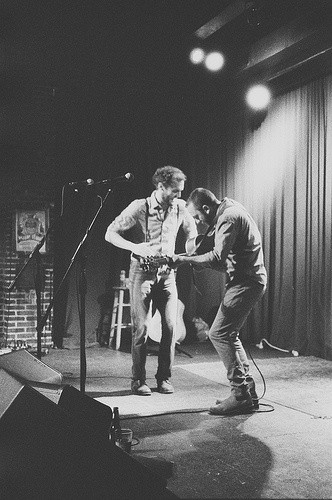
[0,348,181,500]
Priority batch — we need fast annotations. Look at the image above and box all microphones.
[67,178,94,186]
[98,173,135,184]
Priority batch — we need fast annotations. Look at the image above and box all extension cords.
[255,344,264,349]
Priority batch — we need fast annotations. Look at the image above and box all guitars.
[132,229,229,273]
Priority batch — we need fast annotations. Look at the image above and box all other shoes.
[209,394,259,415]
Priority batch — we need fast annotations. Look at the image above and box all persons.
[168,188,267,415]
[105,165,199,396]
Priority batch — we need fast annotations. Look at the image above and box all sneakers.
[131,379,152,395]
[156,376,175,393]
